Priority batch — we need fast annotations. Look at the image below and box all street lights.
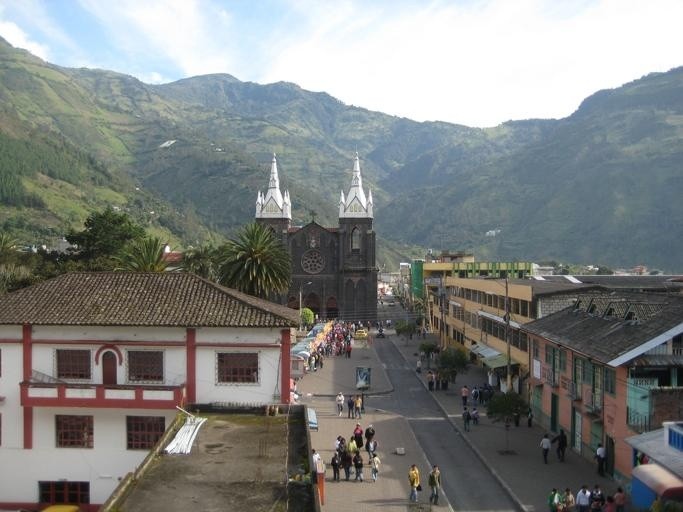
[298,280,313,330]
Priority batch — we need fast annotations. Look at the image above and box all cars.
[352,329,367,340]
[387,300,396,307]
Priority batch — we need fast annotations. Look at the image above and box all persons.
[503,407,533,429]
[310,423,381,483]
[550,429,568,461]
[547,485,626,512]
[428,465,441,505]
[308,318,393,371]
[336,391,362,420]
[596,443,607,473]
[415,347,450,390]
[461,384,495,432]
[407,464,420,503]
[539,434,552,464]
